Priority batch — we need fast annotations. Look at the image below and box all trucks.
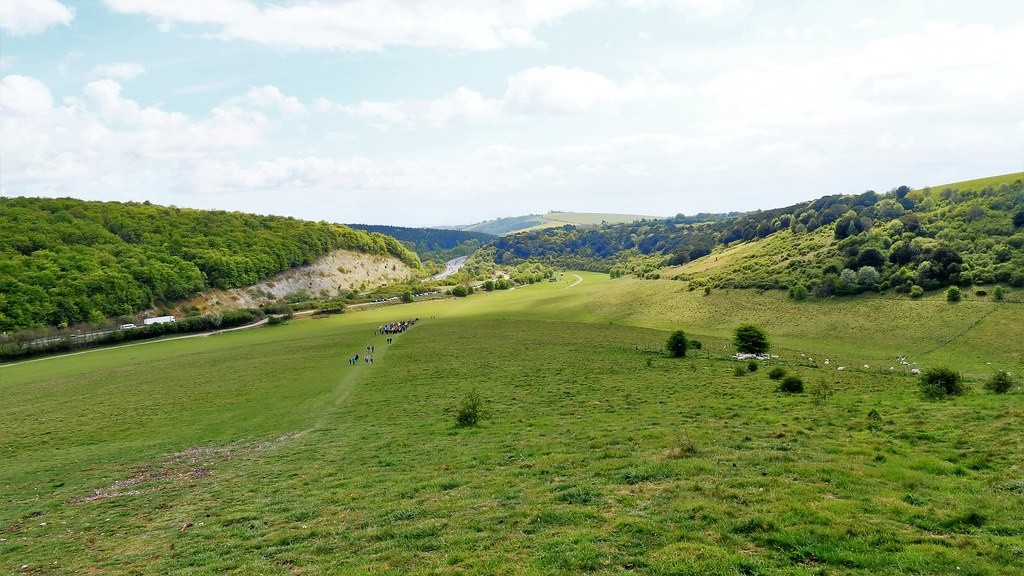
[122,324,137,329]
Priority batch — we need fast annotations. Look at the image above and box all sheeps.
[837,366,845,371]
[808,357,812,361]
[863,364,869,369]
[887,355,921,375]
[800,353,805,357]
[729,351,779,360]
[824,358,830,365]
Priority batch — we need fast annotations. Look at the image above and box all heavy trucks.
[144,316,176,325]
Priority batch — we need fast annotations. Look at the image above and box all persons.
[364,355,368,364]
[370,355,373,363]
[348,352,359,365]
[366,344,370,354]
[386,338,392,344]
[371,345,375,353]
[374,317,418,336]
